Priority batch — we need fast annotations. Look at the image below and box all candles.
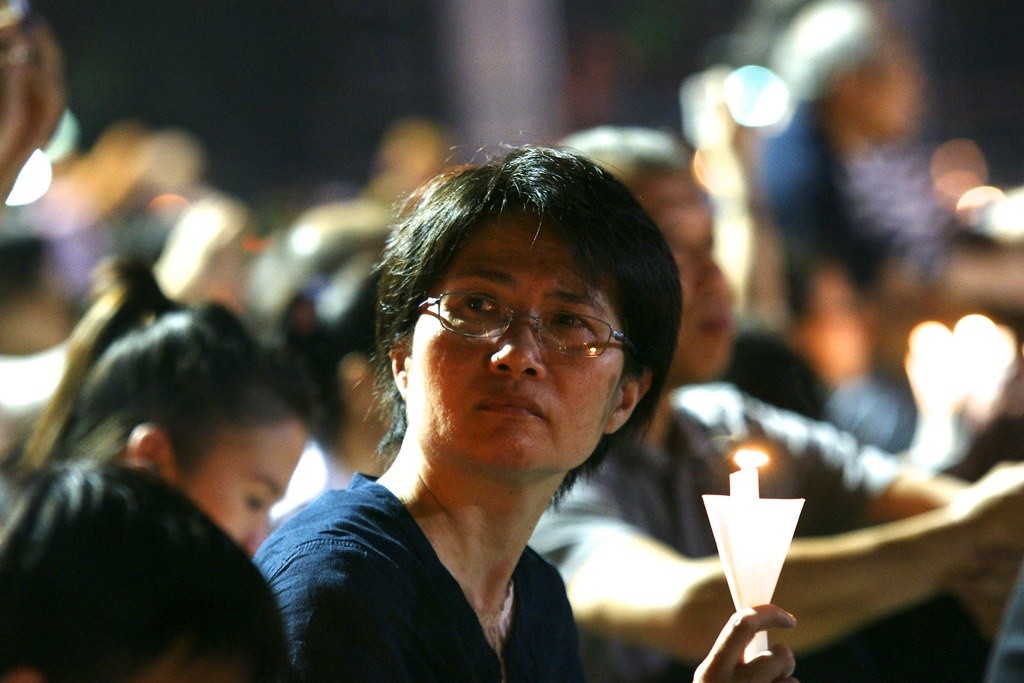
[728,449,768,499]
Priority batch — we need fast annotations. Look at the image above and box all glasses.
[416,291,638,358]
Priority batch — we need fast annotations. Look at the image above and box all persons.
[13,251,309,553]
[720,1,1024,683]
[253,148,802,683]
[0,0,405,560]
[527,126,1024,683]
[0,461,292,683]
